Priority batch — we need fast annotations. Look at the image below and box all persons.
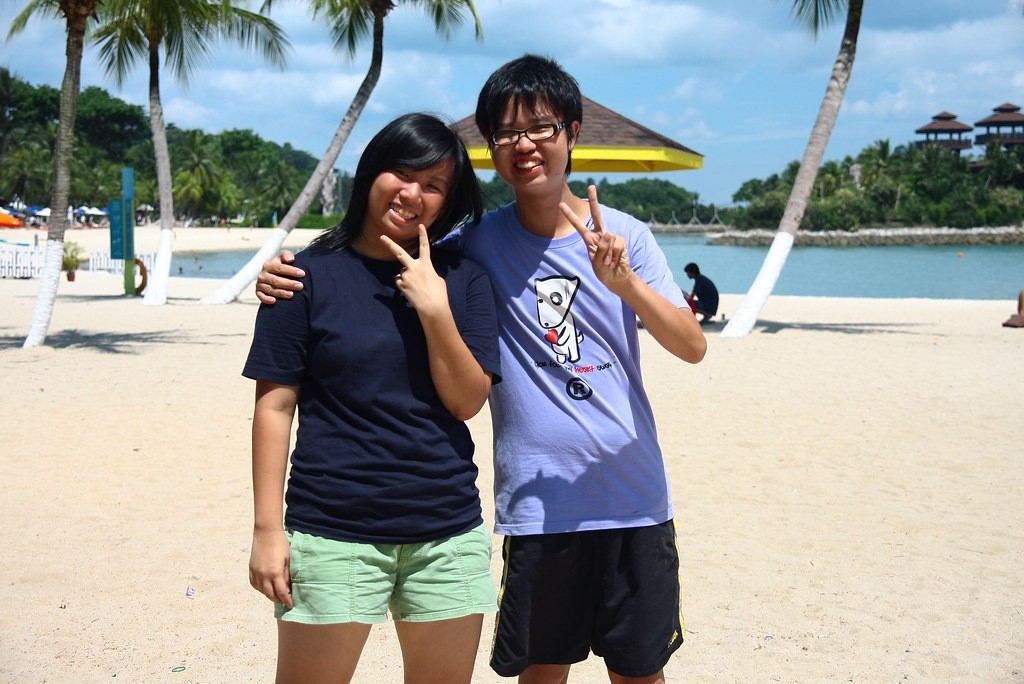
[254,52,707,684]
[682,262,719,324]
[241,113,503,684]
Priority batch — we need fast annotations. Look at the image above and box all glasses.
[487,120,570,146]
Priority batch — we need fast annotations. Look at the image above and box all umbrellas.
[438,94,703,174]
[0,203,153,228]
